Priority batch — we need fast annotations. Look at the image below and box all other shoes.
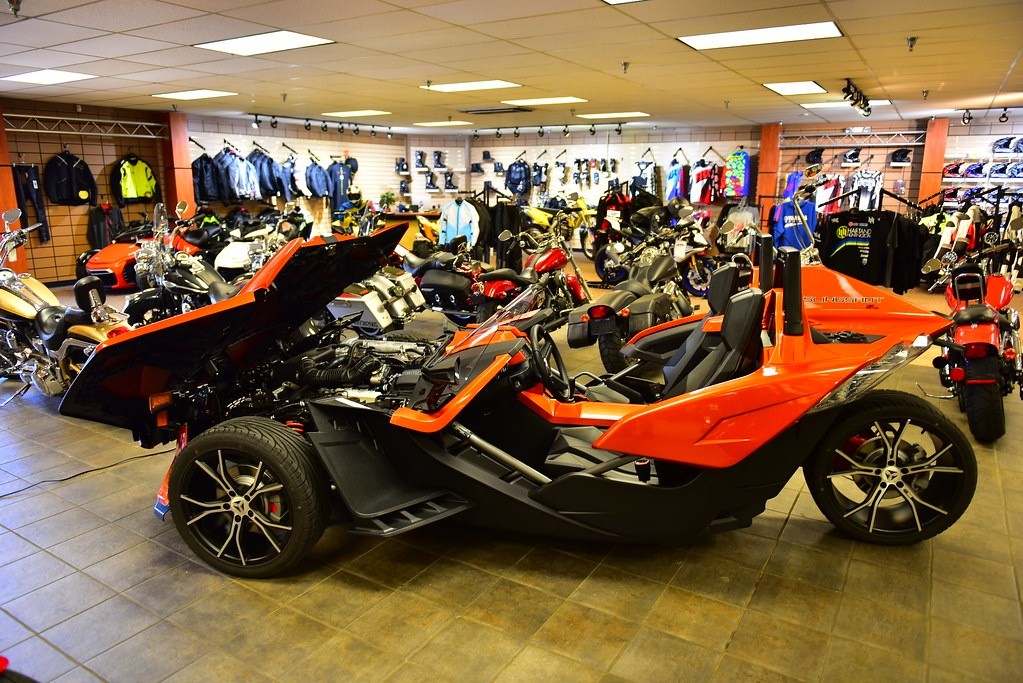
[470,151,507,193]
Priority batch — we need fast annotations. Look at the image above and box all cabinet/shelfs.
[940,150,1023,232]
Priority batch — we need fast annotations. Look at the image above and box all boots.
[433,150,446,168]
[425,171,439,189]
[415,150,427,167]
[444,172,457,189]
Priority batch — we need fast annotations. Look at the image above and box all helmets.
[989,162,1010,178]
[892,148,912,162]
[668,196,690,219]
[843,146,862,163]
[943,187,961,202]
[987,187,1010,203]
[962,187,985,203]
[943,162,965,178]
[347,185,363,206]
[1009,162,1023,178]
[1011,186,1023,202]
[966,163,987,178]
[806,148,824,164]
[992,136,1015,153]
[1013,138,1023,153]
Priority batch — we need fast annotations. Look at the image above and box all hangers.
[455,152,561,204]
[793,164,973,225]
[640,146,748,212]
[13,143,137,210]
[203,137,344,214]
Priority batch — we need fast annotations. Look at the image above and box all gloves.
[573,157,617,186]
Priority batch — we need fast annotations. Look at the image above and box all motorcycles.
[0,181,1023,588]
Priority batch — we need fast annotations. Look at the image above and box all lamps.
[252,114,262,129]
[615,123,622,135]
[589,124,595,135]
[538,126,544,137]
[961,109,973,125]
[271,116,278,128]
[563,125,570,137]
[514,127,520,137]
[999,107,1009,124]
[370,125,376,136]
[338,123,344,133]
[352,124,359,135]
[304,119,311,131]
[841,78,872,118]
[387,127,393,139]
[474,130,479,141]
[321,121,328,132]
[495,128,502,138]
